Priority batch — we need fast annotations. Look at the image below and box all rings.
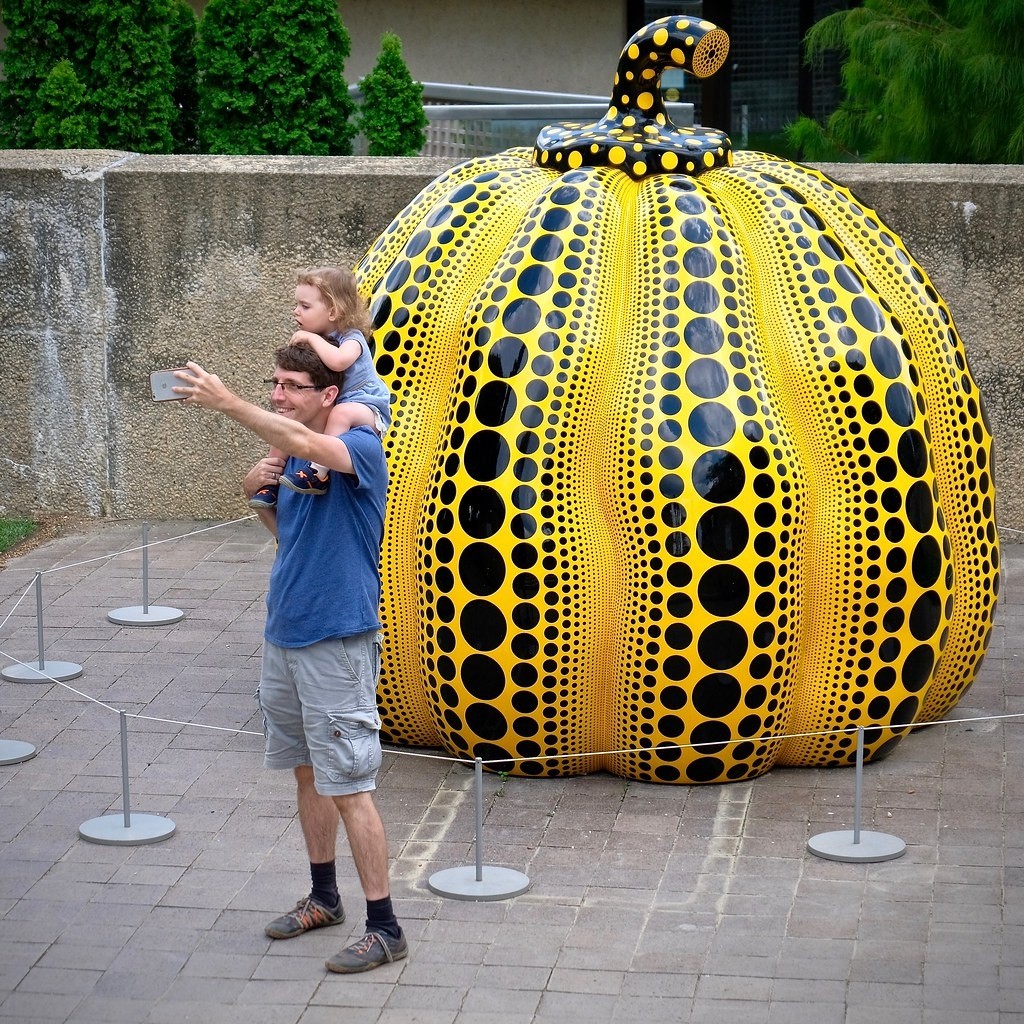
[272,472,276,478]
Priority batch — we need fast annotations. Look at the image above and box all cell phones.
[150,365,204,403]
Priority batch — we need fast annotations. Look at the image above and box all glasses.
[263,379,317,394]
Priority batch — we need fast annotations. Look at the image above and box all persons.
[247,267,390,509]
[172,334,409,974]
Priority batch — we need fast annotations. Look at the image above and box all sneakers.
[264,896,345,938]
[278,462,328,495]
[326,927,407,973]
[248,486,277,510]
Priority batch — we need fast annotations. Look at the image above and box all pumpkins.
[344,15,1002,787]
